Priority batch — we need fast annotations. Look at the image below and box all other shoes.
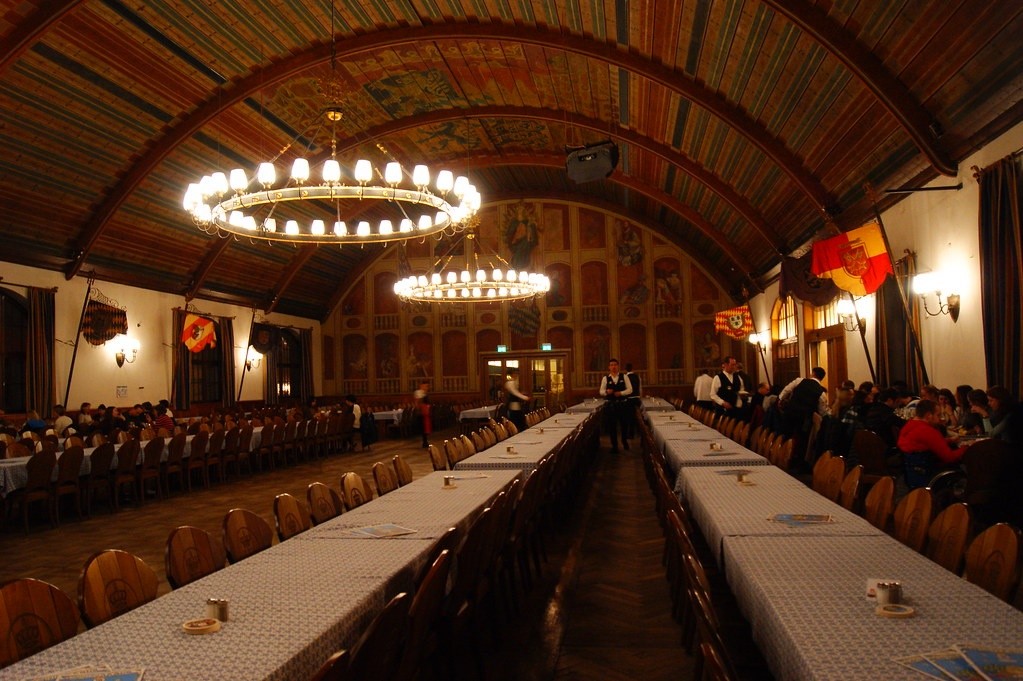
[609,446,619,453]
[622,438,629,450]
[423,442,429,448]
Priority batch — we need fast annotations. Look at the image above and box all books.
[24,664,145,681]
[891,643,1023,681]
[766,512,832,528]
[352,525,417,538]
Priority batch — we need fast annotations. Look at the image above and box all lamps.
[912,271,960,323]
[838,299,866,337]
[182,1,481,250]
[391,119,551,304]
[245,357,261,372]
[114,349,136,368]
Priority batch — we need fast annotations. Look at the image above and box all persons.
[343,392,362,452]
[55,400,177,438]
[504,369,531,434]
[693,369,714,411]
[0,408,46,441]
[507,208,538,269]
[622,269,682,318]
[823,381,1023,560]
[710,356,753,423]
[351,343,432,378]
[599,359,642,454]
[751,382,783,429]
[776,367,828,474]
[302,395,317,420]
[587,329,609,371]
[414,381,431,449]
[617,221,643,267]
[696,332,720,369]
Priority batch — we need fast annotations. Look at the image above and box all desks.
[294,467,526,556]
[663,439,767,473]
[452,426,575,481]
[651,426,723,452]
[372,410,403,437]
[644,411,699,429]
[459,403,499,433]
[722,532,1023,681]
[676,466,880,571]
[567,399,606,429]
[0,540,442,680]
[528,412,591,427]
[1,421,327,517]
[639,398,675,412]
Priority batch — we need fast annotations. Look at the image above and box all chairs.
[0,397,1018,680]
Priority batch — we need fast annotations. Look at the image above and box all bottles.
[737,473,748,481]
[896,407,916,420]
[205,598,229,621]
[646,395,723,451]
[555,419,560,423]
[876,582,902,604]
[507,447,514,453]
[538,428,543,433]
[443,476,455,486]
